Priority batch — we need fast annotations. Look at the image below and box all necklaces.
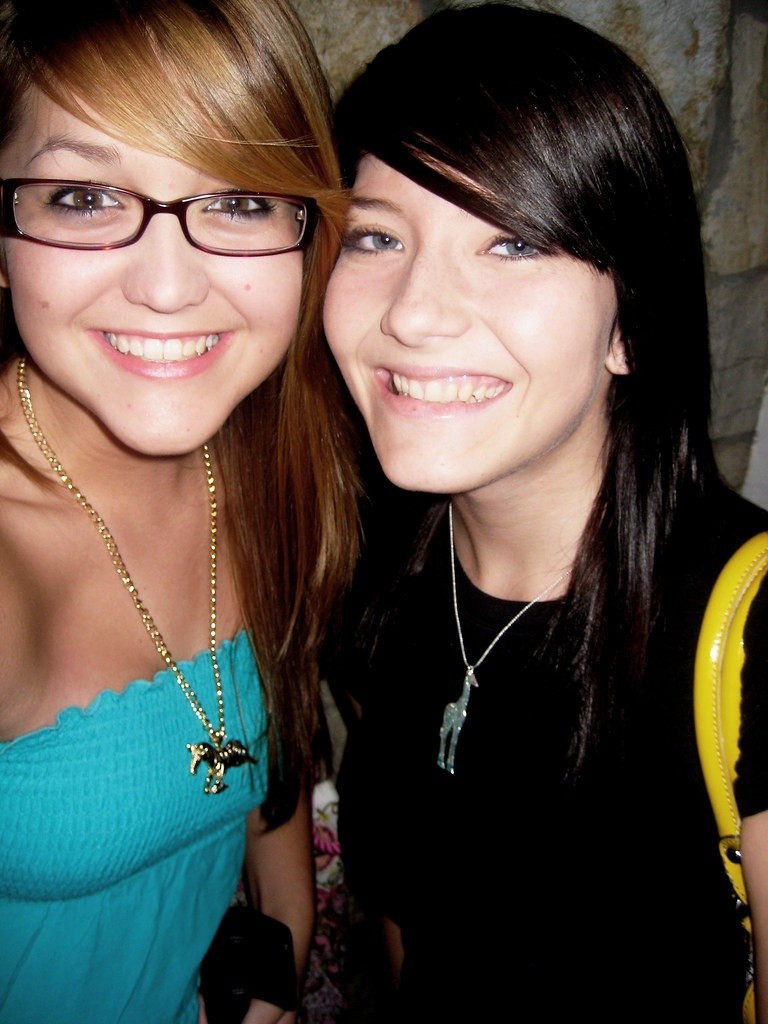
[439,502,571,773]
[18,359,254,790]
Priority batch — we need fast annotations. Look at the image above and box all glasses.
[0,178,318,257]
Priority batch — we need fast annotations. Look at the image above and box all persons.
[0,1,362,1024]
[202,3,768,1023]
